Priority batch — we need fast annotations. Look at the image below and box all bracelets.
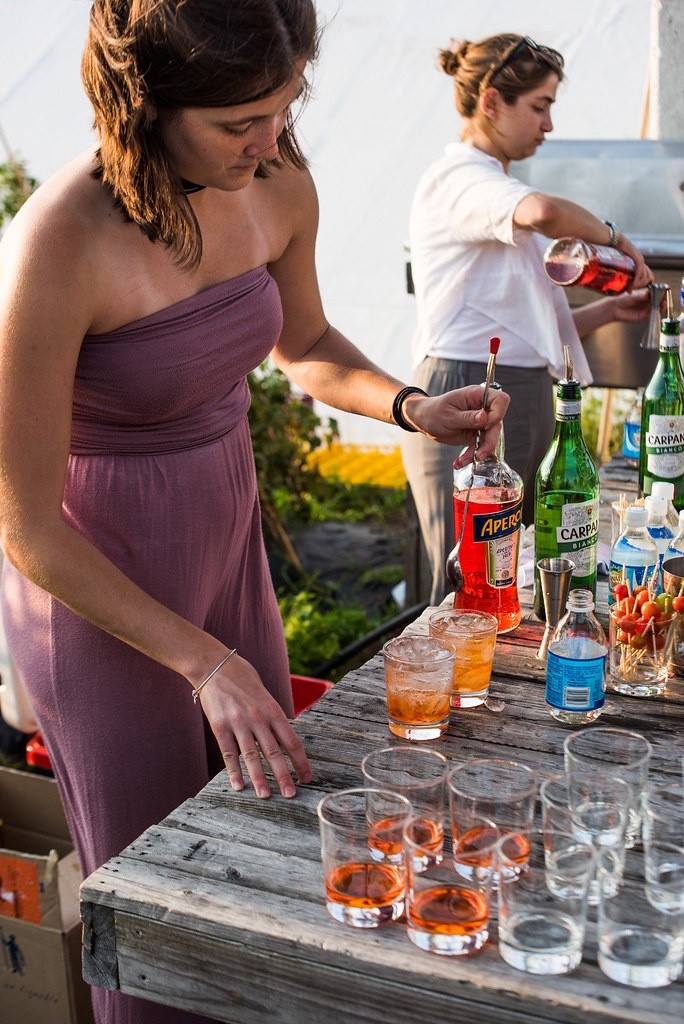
[191,649,236,705]
[605,220,620,248]
[392,386,429,433]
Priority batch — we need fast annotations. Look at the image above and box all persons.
[397,31,667,607]
[0,0,509,1024]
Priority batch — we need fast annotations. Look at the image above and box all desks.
[79,455,684,1024]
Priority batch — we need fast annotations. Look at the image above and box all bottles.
[651,481,678,537]
[677,277,684,373]
[644,495,676,597]
[605,505,663,605]
[663,509,684,561]
[451,357,524,635]
[543,236,651,296]
[637,288,684,512]
[543,589,609,725]
[622,387,645,469]
[533,346,600,622]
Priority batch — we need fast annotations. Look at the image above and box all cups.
[535,557,576,662]
[607,600,679,698]
[316,727,684,991]
[660,556,684,677]
[428,608,498,709]
[639,281,670,350]
[611,505,627,547]
[383,634,458,741]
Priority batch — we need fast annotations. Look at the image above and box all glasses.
[484,36,542,83]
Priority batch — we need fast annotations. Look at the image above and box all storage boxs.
[0,674,335,1024]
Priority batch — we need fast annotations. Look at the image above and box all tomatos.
[611,583,684,651]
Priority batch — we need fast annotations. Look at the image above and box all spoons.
[445,336,500,593]
